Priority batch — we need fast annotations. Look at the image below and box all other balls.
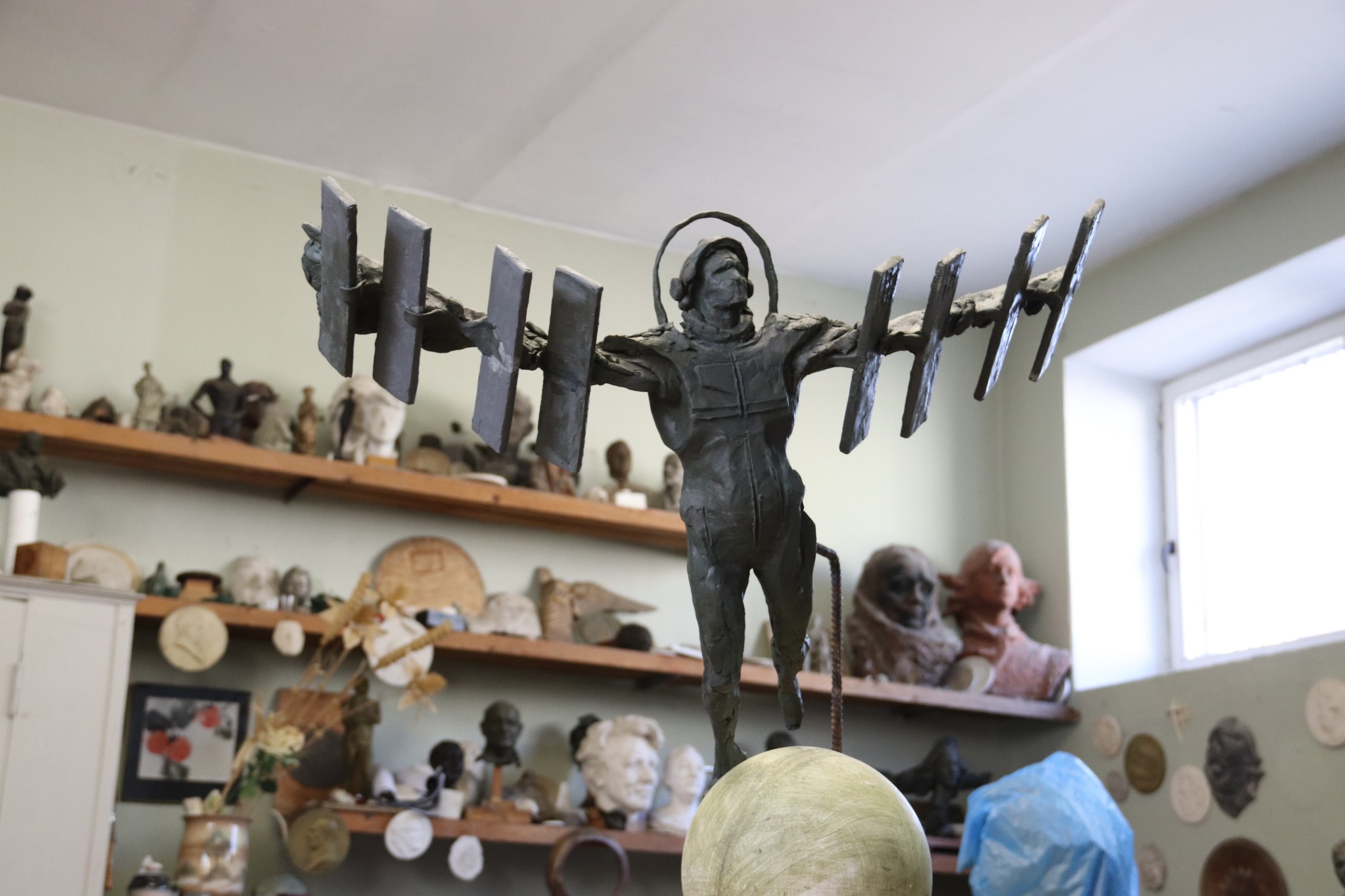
[680,744,933,896]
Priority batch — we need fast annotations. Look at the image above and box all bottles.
[127,859,181,896]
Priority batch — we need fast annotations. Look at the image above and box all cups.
[172,815,252,895]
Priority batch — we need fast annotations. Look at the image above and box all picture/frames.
[120,683,250,805]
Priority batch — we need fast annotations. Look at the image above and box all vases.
[176,814,253,896]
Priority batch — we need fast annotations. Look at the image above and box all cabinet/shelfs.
[0,410,1081,874]
[0,574,146,896]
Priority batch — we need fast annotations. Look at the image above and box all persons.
[308,818,343,871]
[133,361,168,432]
[650,725,715,837]
[473,699,524,767]
[328,366,358,456]
[945,532,1069,699]
[496,390,533,450]
[295,381,318,456]
[341,673,388,796]
[190,351,253,436]
[175,613,215,664]
[845,539,962,689]
[3,284,34,373]
[304,206,1088,778]
[282,566,313,610]
[576,715,667,826]
[605,439,635,480]
[653,450,691,510]
